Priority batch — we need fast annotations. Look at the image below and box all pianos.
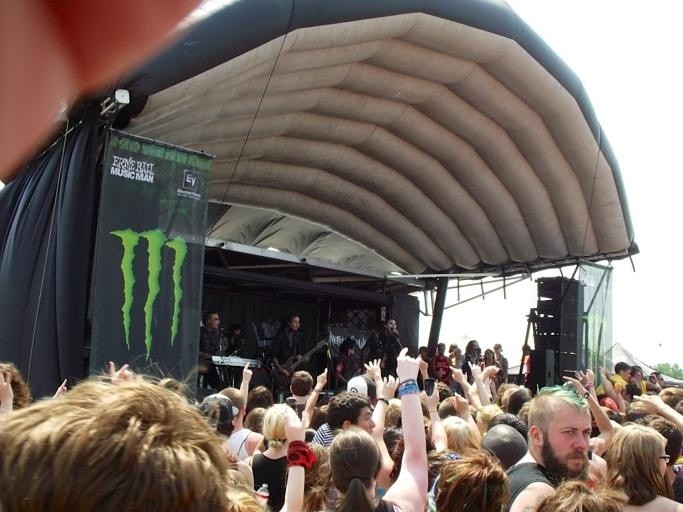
[212,356,260,369]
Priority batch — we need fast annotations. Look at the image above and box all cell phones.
[423,378,435,396]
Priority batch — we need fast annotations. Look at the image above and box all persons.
[0,0,202,185]
[197,312,509,396]
[1,348,682,512]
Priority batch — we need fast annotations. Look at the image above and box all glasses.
[659,455,670,463]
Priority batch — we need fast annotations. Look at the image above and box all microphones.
[220,328,224,337]
[391,328,399,338]
[324,342,332,349]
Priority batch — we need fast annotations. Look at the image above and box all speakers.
[532,349,554,390]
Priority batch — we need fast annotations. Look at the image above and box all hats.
[203,393,239,418]
[482,424,528,469]
[345,375,376,400]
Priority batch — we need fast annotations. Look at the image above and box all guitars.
[271,339,326,391]
